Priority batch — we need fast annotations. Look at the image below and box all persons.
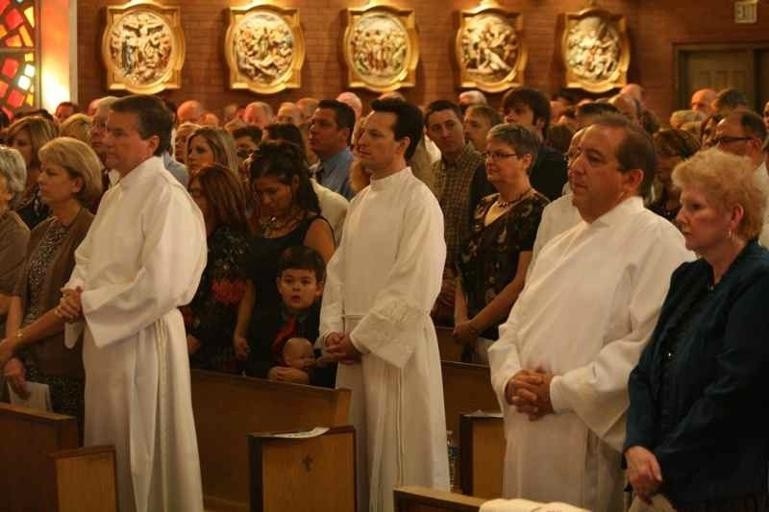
[306,98,362,200]
[567,84,701,137]
[5,116,57,225]
[419,98,482,330]
[233,143,338,384]
[502,85,567,197]
[648,128,702,219]
[0,146,35,319]
[249,244,337,386]
[1,108,54,125]
[55,100,80,126]
[314,96,449,512]
[270,339,315,383]
[89,93,122,191]
[466,103,503,154]
[459,92,485,110]
[701,114,719,150]
[409,107,446,175]
[689,87,717,119]
[186,124,238,178]
[714,112,765,164]
[620,150,767,509]
[713,89,748,118]
[486,118,698,510]
[174,93,320,168]
[52,94,211,495]
[182,163,247,375]
[2,136,105,449]
[448,125,550,362]
[337,93,364,123]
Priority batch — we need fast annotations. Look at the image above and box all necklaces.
[269,209,307,229]
[494,188,531,206]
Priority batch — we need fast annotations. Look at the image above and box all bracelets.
[16,330,24,341]
[467,320,476,331]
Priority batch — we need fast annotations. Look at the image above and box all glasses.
[563,152,579,160]
[714,137,754,143]
[482,151,521,160]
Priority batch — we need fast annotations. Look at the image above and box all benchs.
[187,368,357,511]
[0,407,117,512]
[441,359,508,495]
